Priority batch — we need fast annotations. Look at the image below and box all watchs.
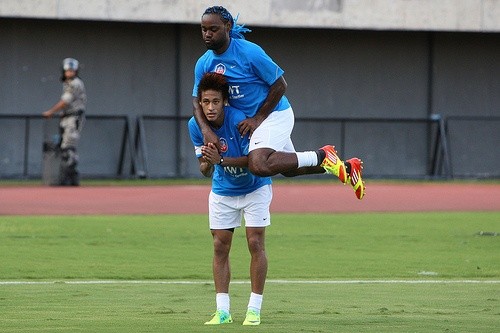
[217,155,224,164]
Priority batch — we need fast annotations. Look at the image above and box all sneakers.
[204,311,233,325]
[346,158,366,201]
[242,311,261,326]
[319,145,349,183]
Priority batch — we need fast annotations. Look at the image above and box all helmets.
[62,58,80,70]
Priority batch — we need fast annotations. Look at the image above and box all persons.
[186,73,272,326]
[191,6,367,199]
[40,56,88,185]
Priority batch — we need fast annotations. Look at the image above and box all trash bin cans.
[41,142,60,183]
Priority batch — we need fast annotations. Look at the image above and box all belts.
[61,110,81,118]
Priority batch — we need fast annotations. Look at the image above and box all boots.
[60,168,80,186]
